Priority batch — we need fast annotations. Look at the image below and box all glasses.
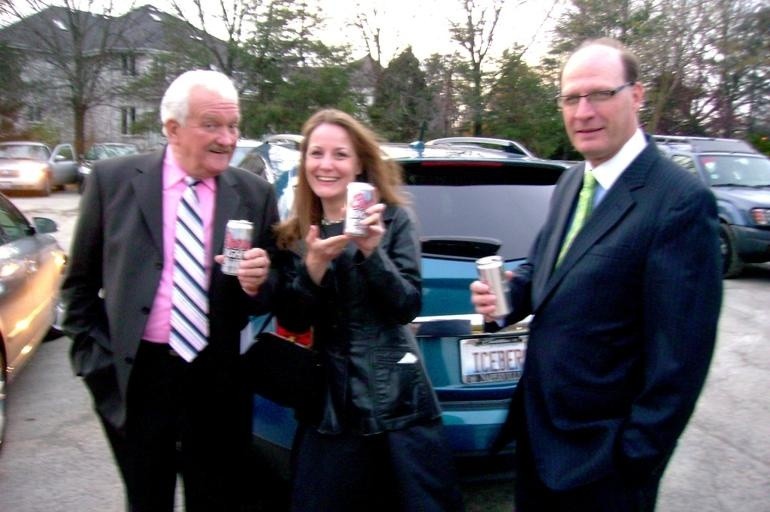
[553,82,634,106]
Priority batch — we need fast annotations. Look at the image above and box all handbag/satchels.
[241,309,330,428]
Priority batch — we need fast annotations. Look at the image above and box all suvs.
[651,134,770,277]
[235,132,573,450]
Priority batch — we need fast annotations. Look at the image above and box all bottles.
[475,255,514,319]
[342,182,377,238]
[221,219,255,277]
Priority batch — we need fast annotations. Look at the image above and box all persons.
[275,107,462,512]
[470,36,724,512]
[59,69,280,512]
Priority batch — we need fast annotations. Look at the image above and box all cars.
[0,141,78,196]
[79,142,136,186]
[0,190,70,456]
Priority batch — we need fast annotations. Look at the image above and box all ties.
[168,175,210,365]
[555,169,598,271]
